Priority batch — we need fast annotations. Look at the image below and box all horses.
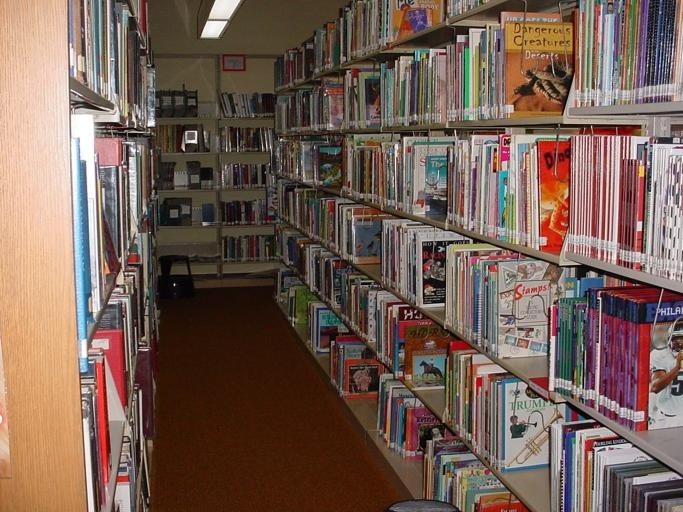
[419,360,443,379]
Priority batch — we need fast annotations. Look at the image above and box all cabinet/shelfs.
[155,118,216,276]
[273,0,683,512]
[0,0,156,511]
[216,117,274,273]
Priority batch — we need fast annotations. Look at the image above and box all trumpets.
[506,407,564,468]
